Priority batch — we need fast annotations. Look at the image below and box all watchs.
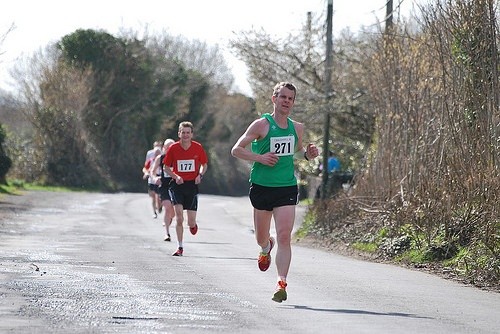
[198,173,203,179]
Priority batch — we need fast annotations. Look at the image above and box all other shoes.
[159,206,162,213]
[154,213,157,218]
[164,234,171,241]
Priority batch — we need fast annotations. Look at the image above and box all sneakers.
[172,249,183,256]
[258,236,276,271]
[189,224,198,235]
[272,280,287,303]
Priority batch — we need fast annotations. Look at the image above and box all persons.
[231,83,318,302]
[163,122,208,256]
[143,139,175,241]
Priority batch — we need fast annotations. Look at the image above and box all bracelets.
[304,152,308,161]
